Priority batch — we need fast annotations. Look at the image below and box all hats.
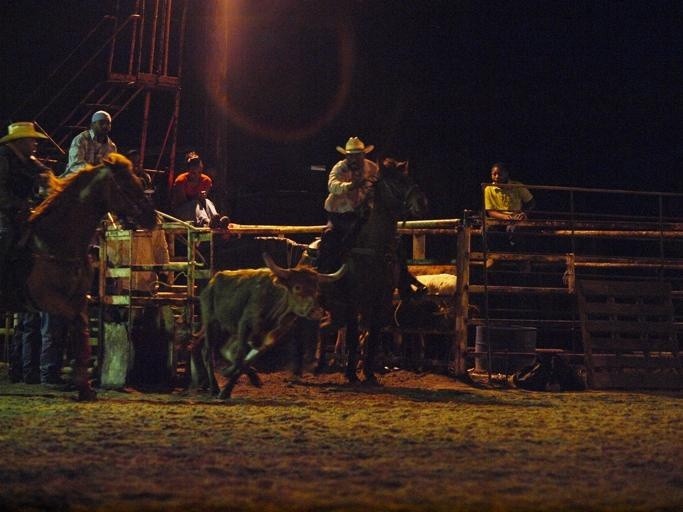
[0,121,49,144]
[336,137,375,156]
[91,111,111,123]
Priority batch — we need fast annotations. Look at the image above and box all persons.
[1,122,55,281]
[481,158,538,269]
[55,110,123,181]
[168,149,231,233]
[123,142,161,230]
[315,135,380,325]
[19,305,73,390]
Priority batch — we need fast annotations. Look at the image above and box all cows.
[200,251,349,399]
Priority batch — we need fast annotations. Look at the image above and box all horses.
[0,154,156,402]
[299,158,429,392]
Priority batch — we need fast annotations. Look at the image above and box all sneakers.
[209,213,230,229]
[6,365,79,391]
